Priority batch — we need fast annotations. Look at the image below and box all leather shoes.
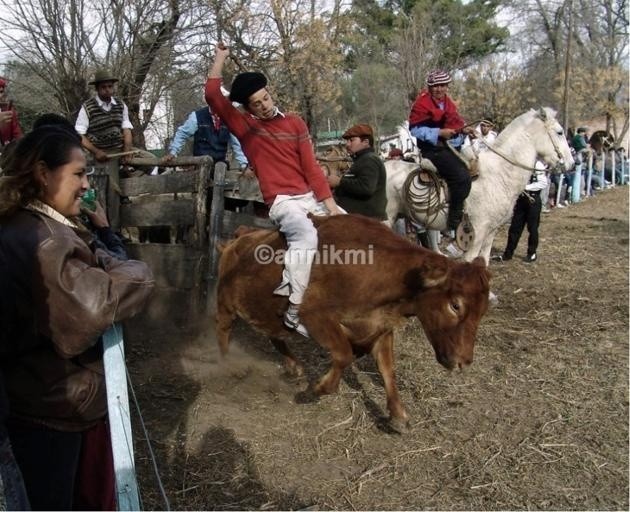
[527,252,537,261]
[448,221,470,230]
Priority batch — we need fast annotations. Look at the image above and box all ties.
[213,113,221,130]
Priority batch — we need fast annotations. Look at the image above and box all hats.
[427,69,452,86]
[342,124,372,139]
[88,70,119,85]
[229,72,267,104]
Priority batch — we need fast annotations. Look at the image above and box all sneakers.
[284,313,309,339]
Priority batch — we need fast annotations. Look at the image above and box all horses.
[585,130,616,191]
[378,106,576,306]
[317,142,353,178]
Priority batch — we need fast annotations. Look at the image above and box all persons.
[476,117,498,143]
[75,70,158,261]
[1,124,155,511]
[323,124,391,231]
[204,41,348,337]
[0,78,25,147]
[161,84,254,180]
[533,125,629,214]
[408,70,480,241]
[495,161,548,262]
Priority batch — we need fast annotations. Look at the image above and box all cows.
[213,212,494,436]
